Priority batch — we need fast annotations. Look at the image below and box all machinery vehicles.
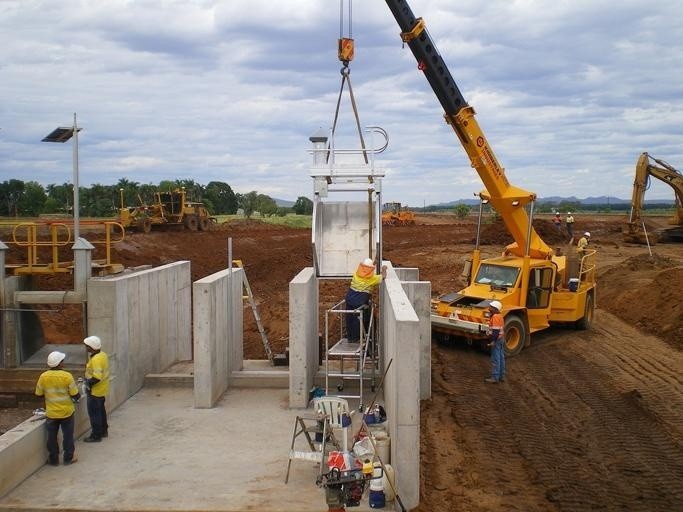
[382,200,416,226]
[113,187,217,233]
[305,0,596,357]
[623,152,683,244]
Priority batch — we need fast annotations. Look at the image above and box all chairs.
[310,398,356,451]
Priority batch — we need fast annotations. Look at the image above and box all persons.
[345,259,388,343]
[487,300,506,382]
[577,232,591,268]
[34,351,81,467]
[81,336,109,442]
[553,212,562,228]
[567,213,573,234]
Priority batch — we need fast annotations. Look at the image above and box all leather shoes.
[484,378,497,383]
[46,458,58,467]
[71,456,78,463]
[102,431,110,437]
[83,436,102,443]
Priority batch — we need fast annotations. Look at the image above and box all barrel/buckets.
[370,430,390,463]
[370,460,396,502]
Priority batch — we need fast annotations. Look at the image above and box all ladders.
[285,413,351,487]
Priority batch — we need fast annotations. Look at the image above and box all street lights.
[41,126,82,243]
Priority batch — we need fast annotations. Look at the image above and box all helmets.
[84,335,102,350]
[490,301,503,313]
[362,258,374,267]
[567,211,571,215]
[555,211,560,216]
[584,231,591,237]
[47,350,66,367]
